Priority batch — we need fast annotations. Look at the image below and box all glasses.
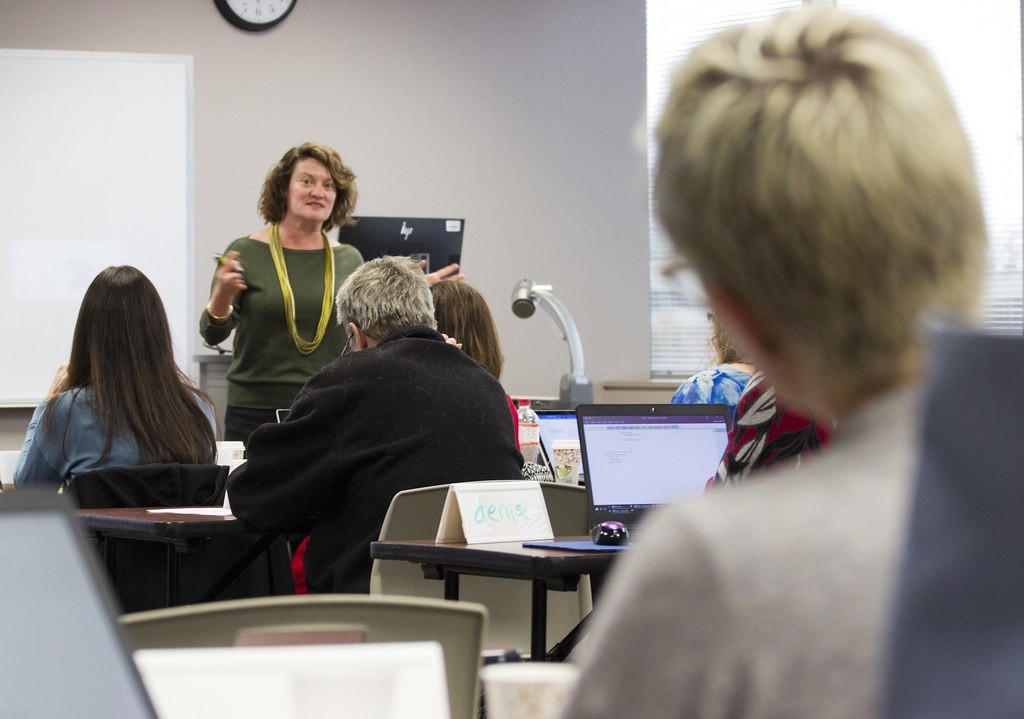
[341,332,354,355]
[664,249,718,302]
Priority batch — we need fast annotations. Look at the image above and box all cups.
[477,661,581,719]
[0,451,19,486]
[216,439,246,510]
[551,440,581,486]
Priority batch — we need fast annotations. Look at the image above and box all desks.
[372,537,630,661]
[73,505,241,611]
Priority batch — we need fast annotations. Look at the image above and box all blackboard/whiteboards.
[0,47,194,410]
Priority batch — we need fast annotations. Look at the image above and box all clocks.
[214,0,297,32]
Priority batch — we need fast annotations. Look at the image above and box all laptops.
[534,404,732,535]
[0,485,161,718]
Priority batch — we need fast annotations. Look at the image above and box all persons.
[14,266,219,493]
[705,369,838,492]
[430,280,521,451]
[199,142,464,458]
[669,317,758,405]
[561,6,989,719]
[226,257,524,597]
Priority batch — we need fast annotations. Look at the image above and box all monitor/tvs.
[338,217,464,280]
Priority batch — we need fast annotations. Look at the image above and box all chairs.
[66,464,584,719]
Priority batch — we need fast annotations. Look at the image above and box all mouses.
[590,521,629,545]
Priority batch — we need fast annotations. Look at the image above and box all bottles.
[514,397,538,465]
